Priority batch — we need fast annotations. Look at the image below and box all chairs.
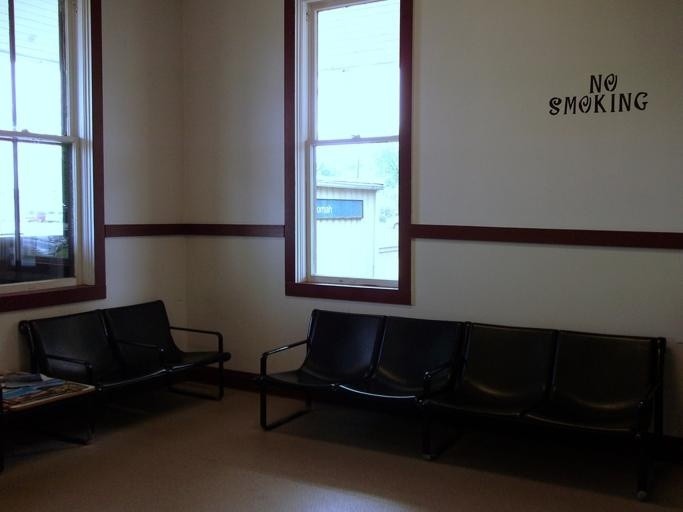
[19,299,231,445]
[253,309,666,501]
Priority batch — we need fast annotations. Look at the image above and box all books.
[0,371,89,407]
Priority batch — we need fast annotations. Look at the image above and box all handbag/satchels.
[422,360,458,398]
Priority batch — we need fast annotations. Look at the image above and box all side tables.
[0,370,98,443]
